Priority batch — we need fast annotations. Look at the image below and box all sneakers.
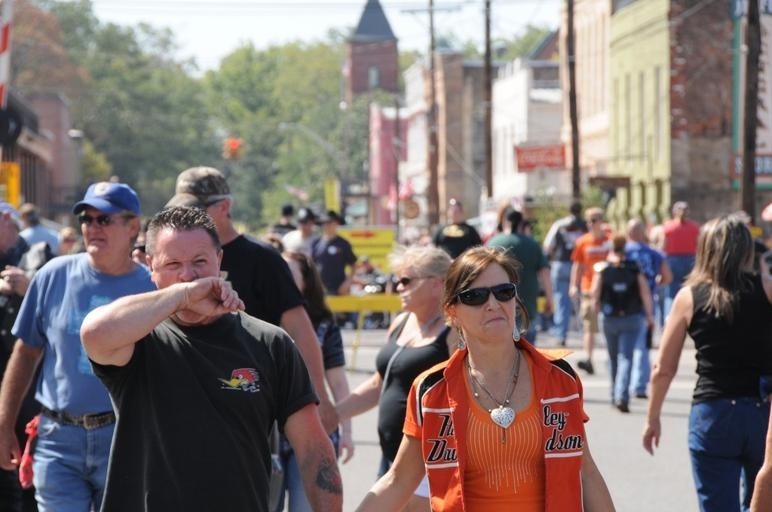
[577,360,594,375]
[614,401,630,413]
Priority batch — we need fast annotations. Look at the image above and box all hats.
[72,179,142,217]
[161,166,233,210]
[295,209,319,221]
[313,210,348,226]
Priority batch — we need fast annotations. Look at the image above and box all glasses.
[449,282,516,306]
[78,214,131,229]
[393,274,439,289]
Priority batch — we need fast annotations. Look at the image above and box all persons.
[591,235,654,413]
[748,403,772,512]
[569,207,613,374]
[663,203,700,298]
[80,207,343,512]
[282,208,319,260]
[17,201,59,258]
[356,248,617,512]
[433,198,484,259]
[333,246,461,480]
[0,181,158,512]
[626,219,672,397]
[271,204,296,235]
[356,259,387,329]
[487,211,554,346]
[541,202,587,347]
[311,210,356,294]
[60,227,77,255]
[644,216,772,512]
[283,251,354,512]
[164,165,341,435]
[0,205,53,373]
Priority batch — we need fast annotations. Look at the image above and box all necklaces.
[466,350,521,443]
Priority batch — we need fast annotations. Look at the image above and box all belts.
[38,406,117,431]
